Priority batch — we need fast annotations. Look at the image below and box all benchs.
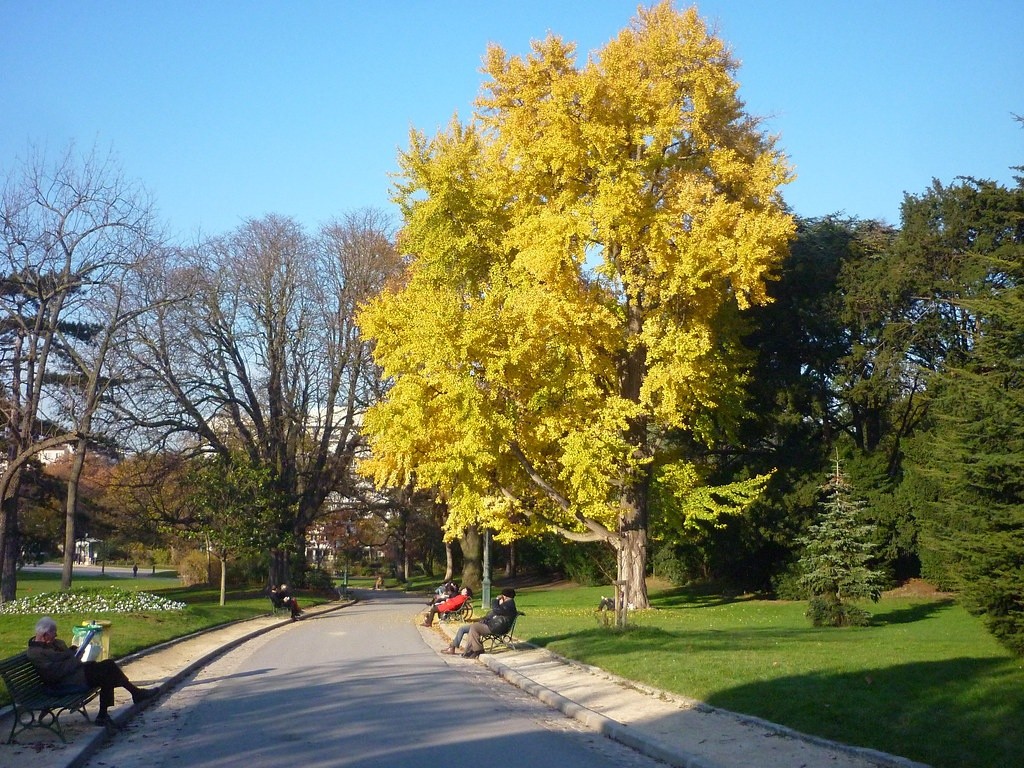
[335,586,351,603]
[267,594,292,618]
[0,643,107,748]
[479,610,527,653]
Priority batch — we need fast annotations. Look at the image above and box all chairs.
[442,594,475,625]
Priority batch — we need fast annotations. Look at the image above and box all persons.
[25,616,160,729]
[440,587,517,658]
[132,565,137,576]
[269,584,303,622]
[420,579,472,627]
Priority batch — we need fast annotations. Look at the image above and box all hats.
[502,588,516,598]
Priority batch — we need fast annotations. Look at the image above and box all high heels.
[94,713,120,729]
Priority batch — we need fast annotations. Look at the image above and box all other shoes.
[133,687,160,704]
[460,650,474,658]
[291,615,298,621]
[420,622,432,627]
[441,614,447,620]
[425,602,432,606]
[441,647,455,654]
[294,613,300,616]
[470,650,485,659]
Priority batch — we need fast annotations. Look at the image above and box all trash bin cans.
[82,620,111,662]
[73,624,102,663]
